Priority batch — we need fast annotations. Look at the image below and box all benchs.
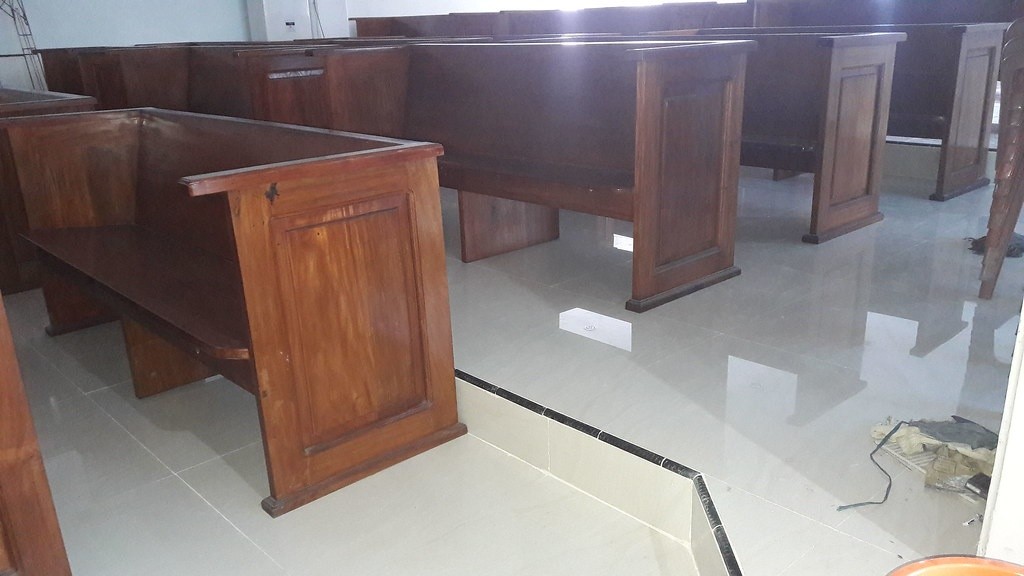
[0,0,1024,576]
